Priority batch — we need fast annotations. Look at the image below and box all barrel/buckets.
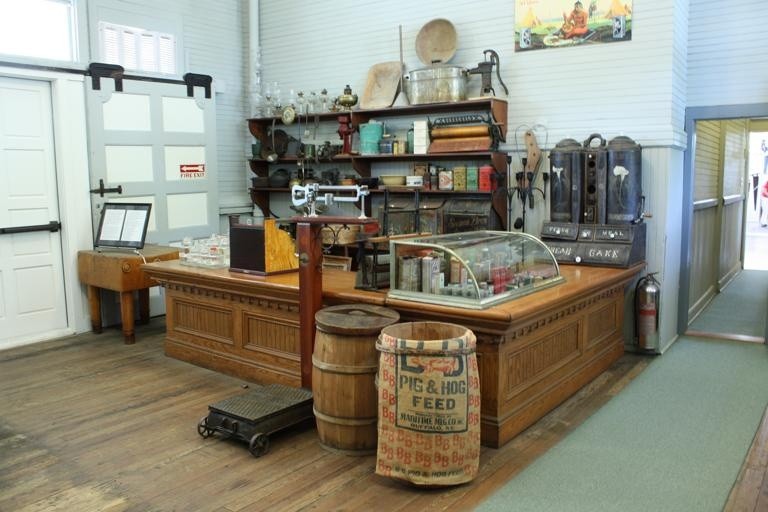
[359,122,383,156]
[311,303,401,455]
[378,320,481,489]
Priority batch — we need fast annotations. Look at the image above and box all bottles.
[183,233,230,270]
[253,45,264,119]
[422,163,500,193]
[377,123,414,155]
[431,248,534,301]
[264,79,339,117]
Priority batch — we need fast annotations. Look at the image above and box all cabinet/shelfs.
[245,102,507,233]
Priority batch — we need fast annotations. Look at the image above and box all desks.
[77,243,180,345]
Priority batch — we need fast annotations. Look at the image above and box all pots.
[404,59,470,106]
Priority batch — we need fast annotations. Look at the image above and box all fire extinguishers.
[634,271,661,356]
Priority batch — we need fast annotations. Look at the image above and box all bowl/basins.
[289,170,406,187]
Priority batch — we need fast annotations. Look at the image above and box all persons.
[561,2,588,40]
[760,180,768,228]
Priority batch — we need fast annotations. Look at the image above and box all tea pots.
[268,171,291,188]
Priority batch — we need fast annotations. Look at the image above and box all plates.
[415,18,459,66]
[359,61,405,110]
